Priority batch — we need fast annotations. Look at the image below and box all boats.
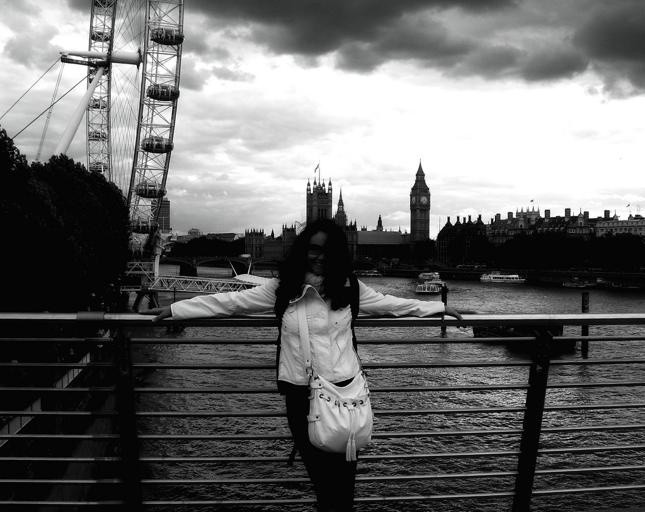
[416,271,441,279]
[356,269,382,278]
[412,279,450,296]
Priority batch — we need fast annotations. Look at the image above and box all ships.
[481,271,527,286]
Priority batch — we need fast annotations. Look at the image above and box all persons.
[140,219,476,512]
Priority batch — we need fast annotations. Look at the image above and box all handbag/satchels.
[308,370,374,454]
